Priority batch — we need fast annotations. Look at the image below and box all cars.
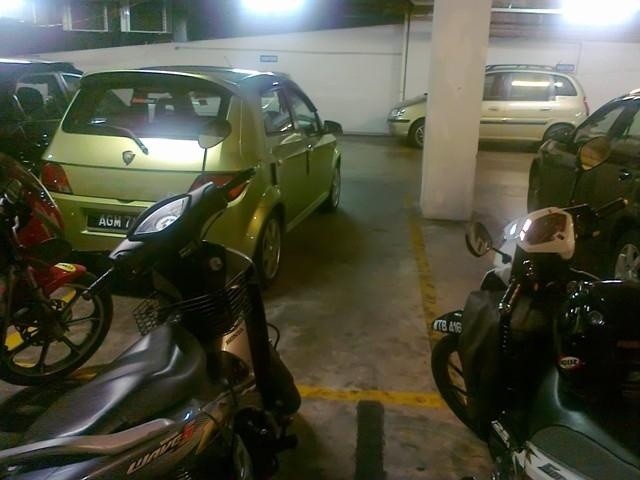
[385,63,592,153]
[39,66,345,293]
[1,60,129,183]
[521,87,640,231]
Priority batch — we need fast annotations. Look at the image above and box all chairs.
[489,75,505,99]
[154,98,190,132]
[16,86,45,120]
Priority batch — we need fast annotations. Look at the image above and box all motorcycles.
[1,118,303,475]
[0,151,112,391]
[429,133,640,480]
